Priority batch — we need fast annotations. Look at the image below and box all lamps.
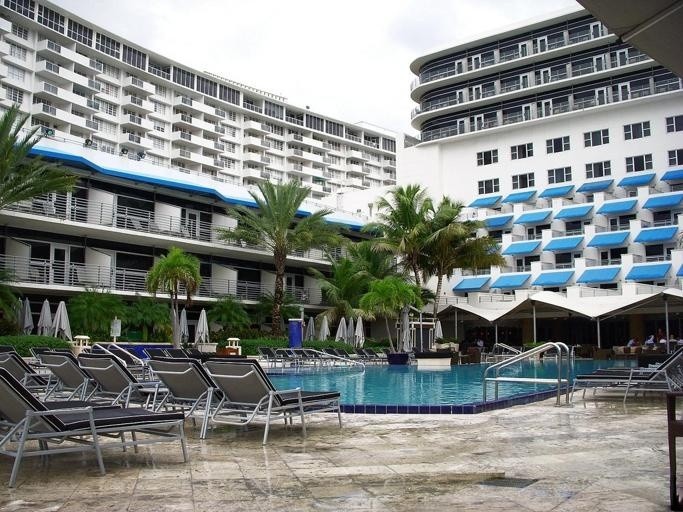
[136,151,146,160]
[119,147,128,156]
[44,128,54,138]
[84,138,93,147]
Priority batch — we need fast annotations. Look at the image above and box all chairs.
[572,339,683,410]
[149,338,343,447]
[0,339,188,478]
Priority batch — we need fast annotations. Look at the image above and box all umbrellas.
[353,316,365,348]
[49,301,73,342]
[318,316,330,342]
[346,317,354,345]
[335,317,348,344]
[22,297,34,336]
[304,316,316,342]
[399,307,413,352]
[178,308,189,343]
[433,321,443,339]
[14,298,23,335]
[194,308,209,354]
[36,299,52,336]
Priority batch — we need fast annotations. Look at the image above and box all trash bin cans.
[73,335,92,356]
[225,337,242,356]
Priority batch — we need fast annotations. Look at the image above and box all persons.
[626,328,682,354]
